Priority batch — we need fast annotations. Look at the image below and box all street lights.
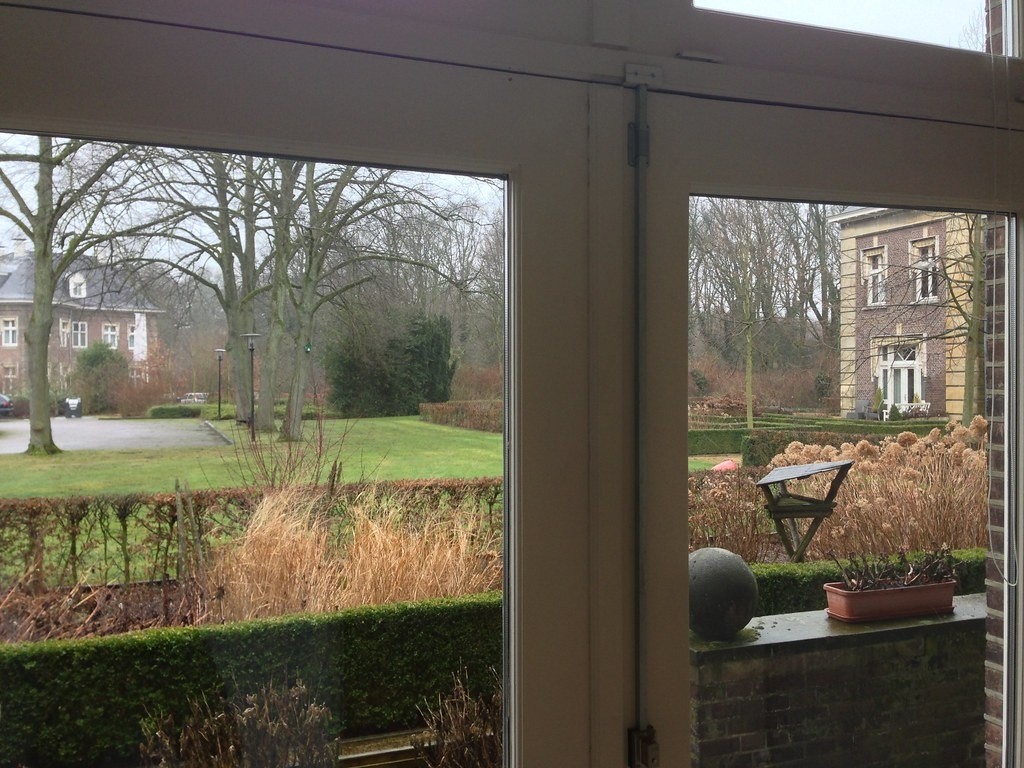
[240,333,263,442]
[214,349,227,422]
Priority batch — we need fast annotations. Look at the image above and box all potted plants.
[822,541,961,623]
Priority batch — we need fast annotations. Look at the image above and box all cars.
[0,394,14,417]
[180,392,207,404]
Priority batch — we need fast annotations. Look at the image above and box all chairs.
[846,399,869,419]
[865,399,884,420]
